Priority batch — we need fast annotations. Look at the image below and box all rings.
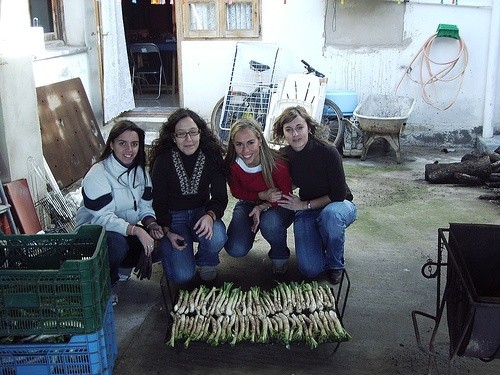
[151,244,153,247]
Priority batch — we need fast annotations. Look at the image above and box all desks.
[136,36,178,95]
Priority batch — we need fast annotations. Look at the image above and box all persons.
[73,118,164,282]
[224,117,296,284]
[272,105,358,283]
[147,107,229,285]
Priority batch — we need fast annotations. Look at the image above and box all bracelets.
[147,222,157,229]
[263,203,269,212]
[207,211,216,221]
[131,225,135,235]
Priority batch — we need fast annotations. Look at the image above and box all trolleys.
[352,94,417,163]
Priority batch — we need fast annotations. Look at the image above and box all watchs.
[306,199,312,210]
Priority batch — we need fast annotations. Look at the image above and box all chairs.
[129,43,169,100]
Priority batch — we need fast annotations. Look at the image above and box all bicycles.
[211,59,345,150]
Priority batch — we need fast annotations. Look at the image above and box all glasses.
[174,128,202,138]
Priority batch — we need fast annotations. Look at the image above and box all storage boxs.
[0,224,111,335]
[0,292,118,375]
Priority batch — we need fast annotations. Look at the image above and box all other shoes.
[111,281,120,307]
[272,258,291,280]
[328,268,342,284]
[119,266,133,281]
[201,264,217,280]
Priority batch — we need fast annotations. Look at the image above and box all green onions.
[166,281,350,349]
[0,296,85,375]
